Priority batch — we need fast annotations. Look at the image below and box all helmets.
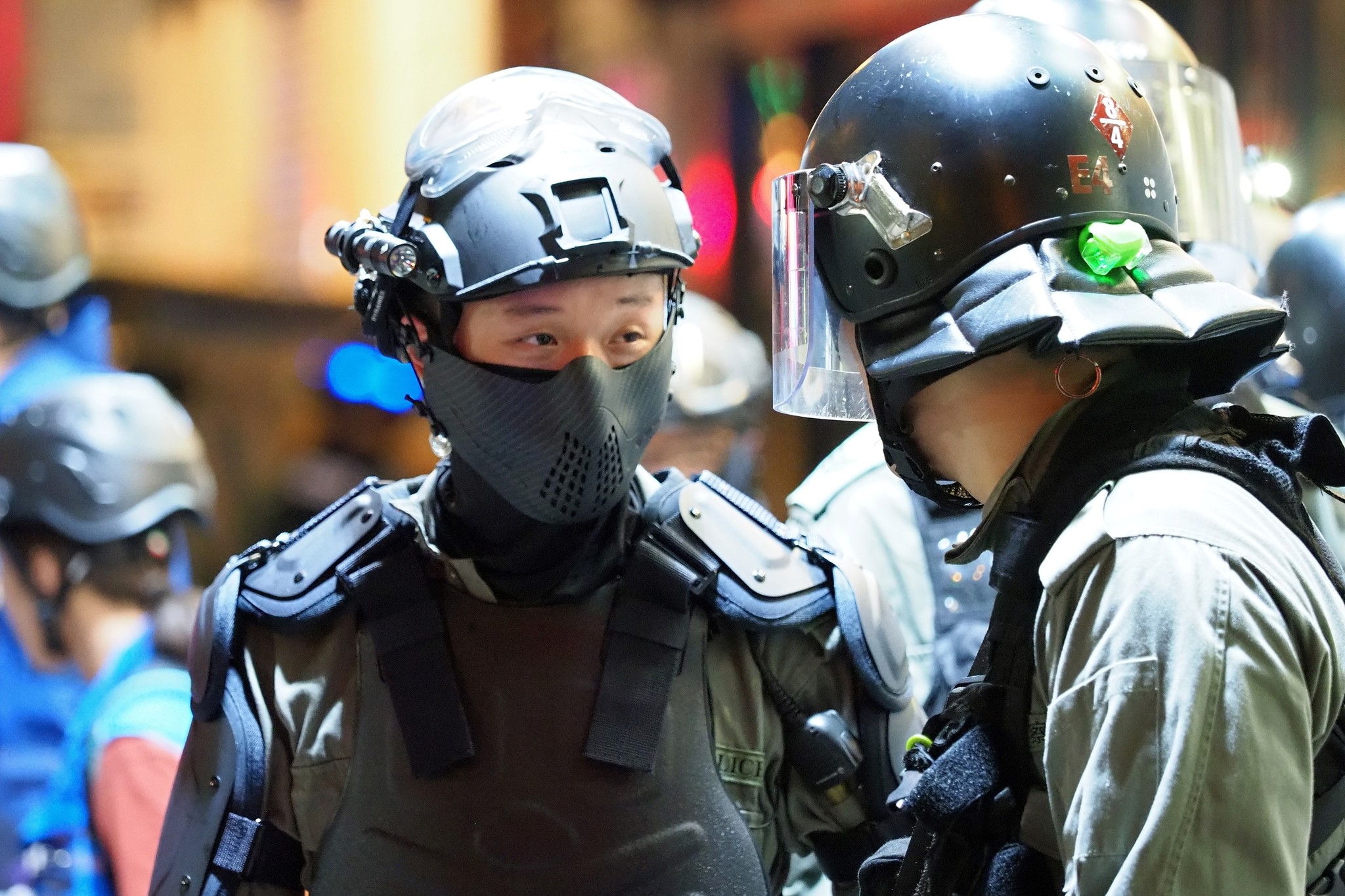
[0,142,95,309]
[0,367,221,543]
[1250,227,1345,421]
[658,291,770,423]
[771,11,1176,424]
[380,62,700,304]
[965,0,1253,262]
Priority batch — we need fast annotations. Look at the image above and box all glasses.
[389,66,681,240]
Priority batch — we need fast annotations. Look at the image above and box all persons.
[638,290,770,504]
[145,66,928,896]
[1238,194,1345,448]
[0,370,217,896]
[251,343,436,538]
[771,17,1345,895]
[0,140,111,418]
[781,419,999,894]
